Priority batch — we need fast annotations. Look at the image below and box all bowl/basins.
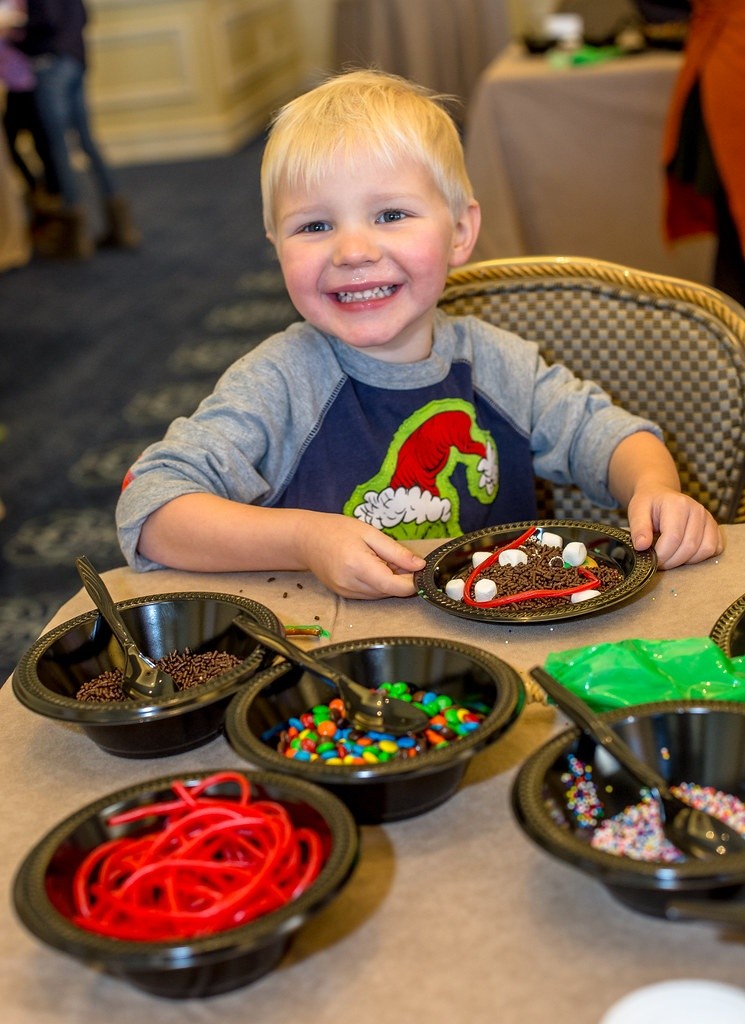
[510,699,745,921]
[10,591,286,759]
[709,592,745,659]
[10,769,361,1000]
[224,636,528,825]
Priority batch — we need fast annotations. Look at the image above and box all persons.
[0,1,134,261]
[115,72,725,600]
[660,0,745,310]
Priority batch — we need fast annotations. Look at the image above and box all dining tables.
[458,30,718,294]
[0,523,745,1024]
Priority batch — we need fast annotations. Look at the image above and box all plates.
[413,519,658,623]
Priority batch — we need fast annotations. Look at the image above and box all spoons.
[75,556,180,703]
[233,615,428,733]
[530,667,745,863]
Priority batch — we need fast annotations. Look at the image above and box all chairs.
[437,255,745,525]
